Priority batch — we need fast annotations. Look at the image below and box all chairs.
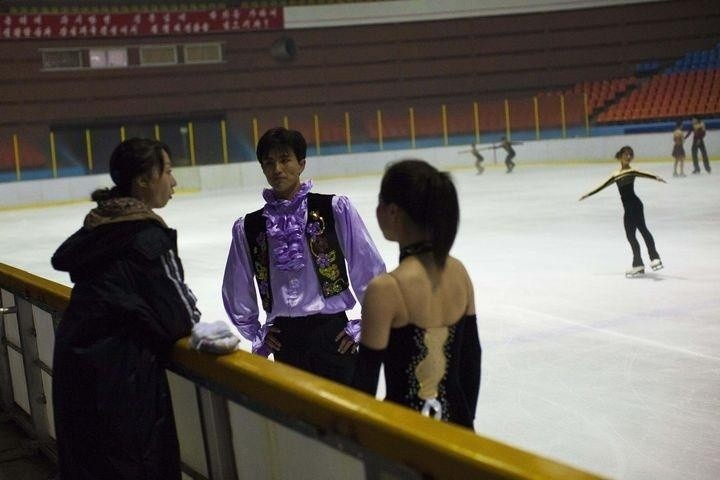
[600,50,720,124]
[541,81,600,115]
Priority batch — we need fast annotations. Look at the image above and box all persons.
[221,125,387,398]
[457,143,491,175]
[349,159,482,435]
[684,115,711,174]
[51,139,202,480]
[672,119,686,178]
[488,136,524,174]
[578,145,667,279]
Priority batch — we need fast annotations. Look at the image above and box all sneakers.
[650,259,662,268]
[626,266,644,274]
